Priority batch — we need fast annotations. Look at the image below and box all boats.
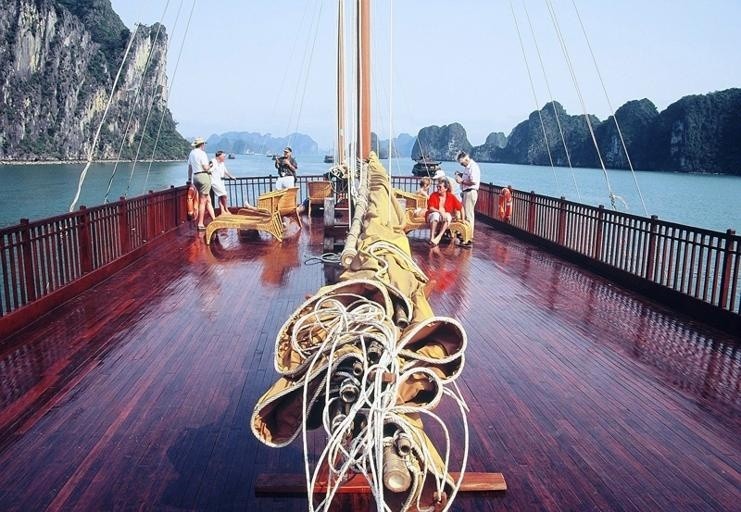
[322,153,335,163]
[408,151,444,178]
[222,147,282,161]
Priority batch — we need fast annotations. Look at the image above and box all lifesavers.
[187,186,199,223]
[498,188,512,223]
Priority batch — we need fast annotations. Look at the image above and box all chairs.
[204,175,475,250]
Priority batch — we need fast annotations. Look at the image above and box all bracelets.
[188,178,191,180]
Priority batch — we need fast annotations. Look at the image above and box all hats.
[434,170,446,180]
[283,146,293,153]
[191,137,208,147]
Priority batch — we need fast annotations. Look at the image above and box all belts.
[463,189,479,192]
[193,171,208,175]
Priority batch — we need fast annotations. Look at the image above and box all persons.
[455,151,481,248]
[297,197,310,215]
[416,175,432,200]
[432,170,463,203]
[186,137,217,231]
[211,151,237,215]
[247,202,260,211]
[426,179,465,246]
[275,147,298,191]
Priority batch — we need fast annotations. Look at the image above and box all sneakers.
[197,225,207,230]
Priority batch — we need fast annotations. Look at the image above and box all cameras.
[455,171,463,178]
